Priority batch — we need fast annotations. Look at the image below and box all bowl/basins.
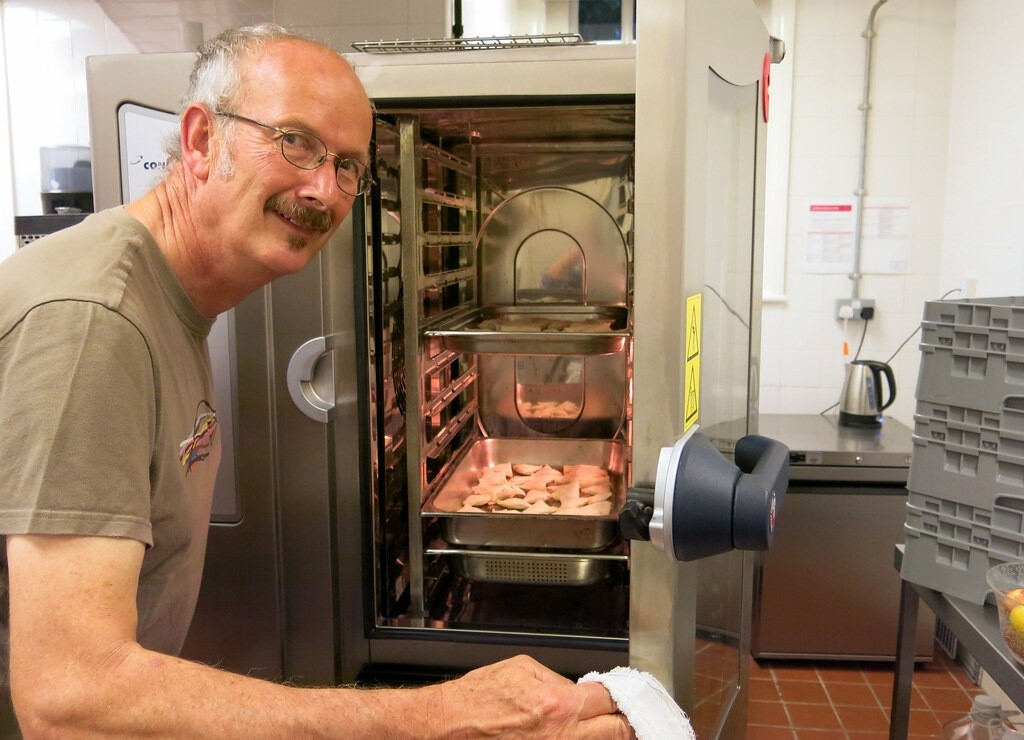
[985,561,1024,665]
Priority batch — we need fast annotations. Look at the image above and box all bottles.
[936,691,1024,740]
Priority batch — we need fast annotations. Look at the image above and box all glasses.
[214,113,378,198]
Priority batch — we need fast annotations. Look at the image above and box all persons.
[0,23,637,740]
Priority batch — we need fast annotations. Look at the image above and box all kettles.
[838,360,897,429]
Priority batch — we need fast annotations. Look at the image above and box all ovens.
[78,21,791,739]
